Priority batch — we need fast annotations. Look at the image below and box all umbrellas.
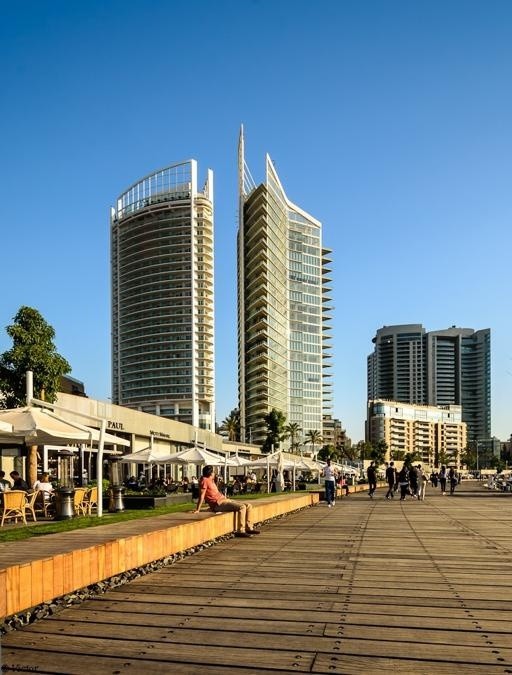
[1,371,131,518]
[119,432,353,496]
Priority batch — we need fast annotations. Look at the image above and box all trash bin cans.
[108,485,125,512]
[52,488,75,518]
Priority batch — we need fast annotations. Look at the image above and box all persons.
[192,476,198,483]
[337,471,351,495]
[430,465,463,494]
[482,474,512,492]
[182,477,189,485]
[385,462,396,500]
[304,473,314,482]
[9,471,31,504]
[215,475,243,492]
[243,471,257,483]
[35,473,54,509]
[190,464,260,539]
[139,471,146,490]
[322,458,337,508]
[394,459,429,500]
[0,471,12,506]
[367,462,377,499]
[162,477,172,486]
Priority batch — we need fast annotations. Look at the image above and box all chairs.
[165,481,255,503]
[0,487,98,526]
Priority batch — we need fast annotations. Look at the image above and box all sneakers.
[246,528,260,534]
[235,532,248,538]
